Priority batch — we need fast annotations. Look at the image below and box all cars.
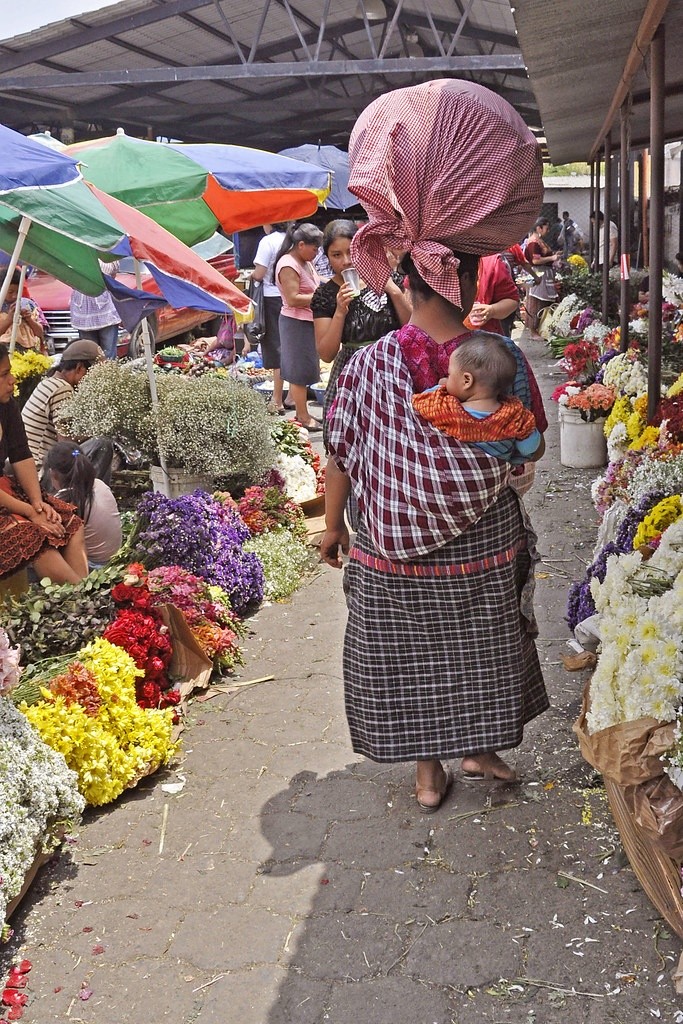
[28,231,242,365]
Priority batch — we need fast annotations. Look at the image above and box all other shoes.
[295,413,324,431]
[529,333,545,342]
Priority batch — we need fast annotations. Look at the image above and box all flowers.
[544,256,683,806]
[0,346,325,944]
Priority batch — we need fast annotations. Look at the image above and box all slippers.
[282,401,298,411]
[266,406,285,415]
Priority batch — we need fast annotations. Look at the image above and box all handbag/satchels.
[244,276,265,345]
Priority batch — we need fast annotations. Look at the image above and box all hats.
[62,339,106,369]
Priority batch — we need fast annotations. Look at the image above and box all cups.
[340,268,361,297]
[468,302,481,326]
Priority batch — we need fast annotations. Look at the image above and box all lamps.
[400,33,425,58]
[355,0,387,21]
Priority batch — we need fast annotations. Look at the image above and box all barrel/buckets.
[149,464,213,498]
[558,405,608,468]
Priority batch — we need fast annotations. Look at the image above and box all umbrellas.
[275,138,359,212]
[0,231,234,359]
[56,127,335,498]
[0,122,259,314]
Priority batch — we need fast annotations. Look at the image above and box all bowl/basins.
[310,383,331,405]
[303,386,316,399]
[252,384,299,400]
[162,354,186,362]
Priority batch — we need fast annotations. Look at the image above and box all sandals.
[414,763,455,813]
[459,753,523,789]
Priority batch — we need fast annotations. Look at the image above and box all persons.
[0,266,260,602]
[523,211,619,336]
[638,252,683,303]
[254,220,551,809]
